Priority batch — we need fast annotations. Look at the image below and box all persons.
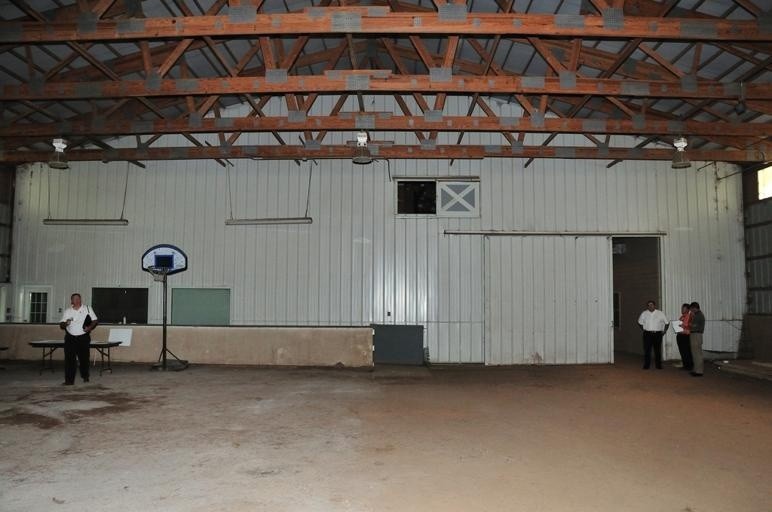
[59,293,98,385]
[676,303,693,370]
[637,300,670,369]
[688,302,705,377]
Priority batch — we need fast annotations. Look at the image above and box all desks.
[0,346,10,372]
[27,341,122,376]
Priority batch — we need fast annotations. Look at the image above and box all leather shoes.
[62,383,74,385]
[84,378,89,382]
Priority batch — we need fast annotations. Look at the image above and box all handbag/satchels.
[82,314,91,333]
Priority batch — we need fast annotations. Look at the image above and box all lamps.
[49,138,70,170]
[671,137,691,169]
[352,131,373,164]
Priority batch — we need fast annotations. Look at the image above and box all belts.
[691,331,702,333]
[647,331,662,334]
